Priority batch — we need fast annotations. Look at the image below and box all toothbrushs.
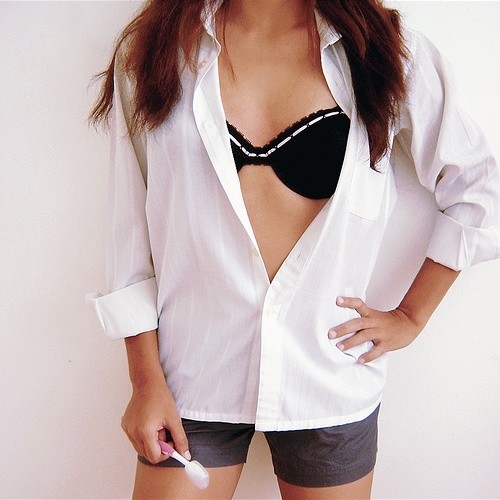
[157,439,210,490]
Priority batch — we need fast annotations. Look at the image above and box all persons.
[88,0,499,500]
